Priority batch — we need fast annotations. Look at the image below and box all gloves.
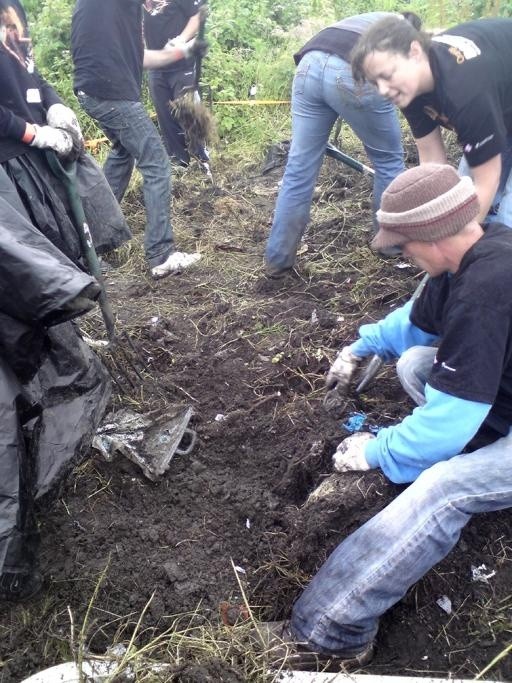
[27,122,75,156]
[331,430,377,474]
[182,36,208,61]
[326,346,363,396]
[163,34,185,49]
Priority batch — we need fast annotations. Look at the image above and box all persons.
[253,8,424,283]
[243,161,512,674]
[69,0,204,281]
[141,0,213,185]
[348,9,511,228]
[0,0,99,393]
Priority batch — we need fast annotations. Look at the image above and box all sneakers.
[151,250,202,280]
[249,619,375,671]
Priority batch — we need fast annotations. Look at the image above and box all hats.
[370,162,482,250]
